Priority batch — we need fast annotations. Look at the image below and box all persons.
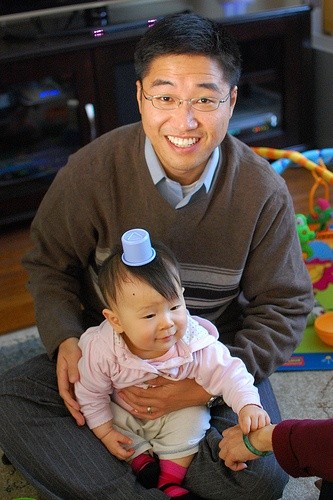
[74,228,272,498]
[0,9,313,500]
[218,418,333,479]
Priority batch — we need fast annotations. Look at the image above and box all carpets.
[0,325,333,500]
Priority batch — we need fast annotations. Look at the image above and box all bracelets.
[241,432,273,457]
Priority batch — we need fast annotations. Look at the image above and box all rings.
[147,406,152,415]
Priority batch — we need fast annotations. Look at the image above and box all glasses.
[140,81,231,112]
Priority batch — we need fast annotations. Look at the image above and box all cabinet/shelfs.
[0,0,314,233]
